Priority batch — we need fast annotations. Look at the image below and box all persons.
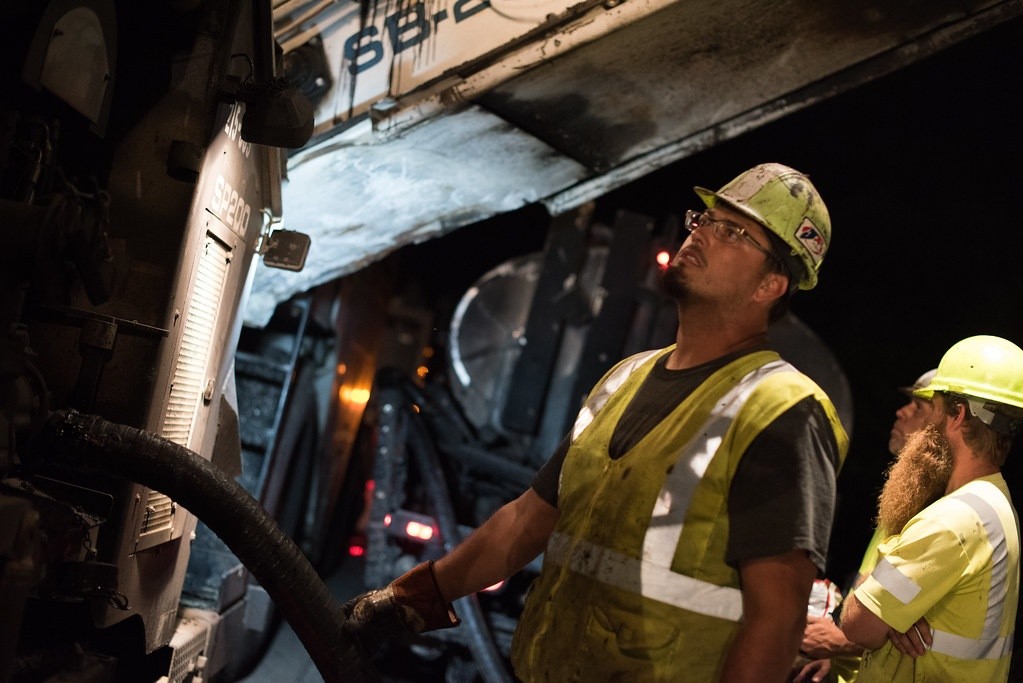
[344,162,850,683]
[841,335,1023,683]
[793,369,938,683]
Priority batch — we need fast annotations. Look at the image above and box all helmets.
[901,368,936,401]
[694,163,831,290]
[919,335,1023,408]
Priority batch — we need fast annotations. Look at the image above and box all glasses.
[685,210,787,274]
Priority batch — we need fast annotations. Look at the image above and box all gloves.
[339,561,461,661]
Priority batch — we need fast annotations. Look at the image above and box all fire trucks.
[0,0,679,681]
